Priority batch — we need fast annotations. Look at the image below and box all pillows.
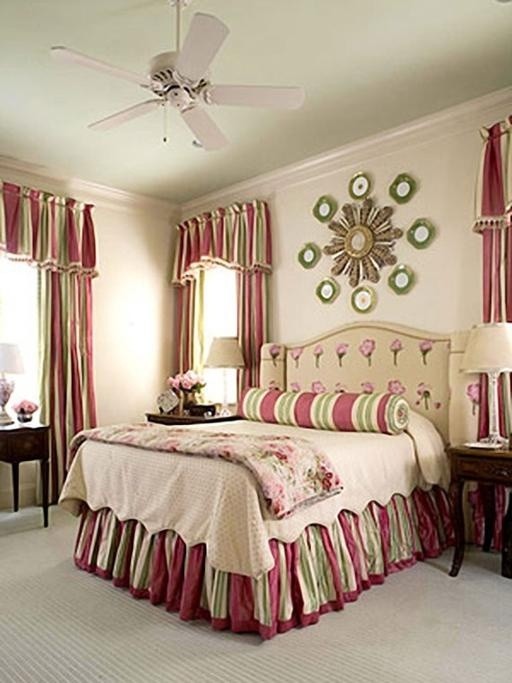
[238,386,411,435]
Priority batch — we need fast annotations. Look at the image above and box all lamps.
[0,341,25,426]
[459,322,512,448]
[202,335,247,415]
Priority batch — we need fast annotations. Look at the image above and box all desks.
[0,423,53,527]
[445,443,511,577]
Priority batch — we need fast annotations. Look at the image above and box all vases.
[179,389,198,415]
[16,413,32,422]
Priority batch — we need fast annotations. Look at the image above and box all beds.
[80,319,480,635]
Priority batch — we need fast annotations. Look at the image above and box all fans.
[47,0,306,151]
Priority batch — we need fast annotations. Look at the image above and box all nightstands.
[144,410,240,425]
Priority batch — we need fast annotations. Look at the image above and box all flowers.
[166,369,207,394]
[11,399,38,414]
